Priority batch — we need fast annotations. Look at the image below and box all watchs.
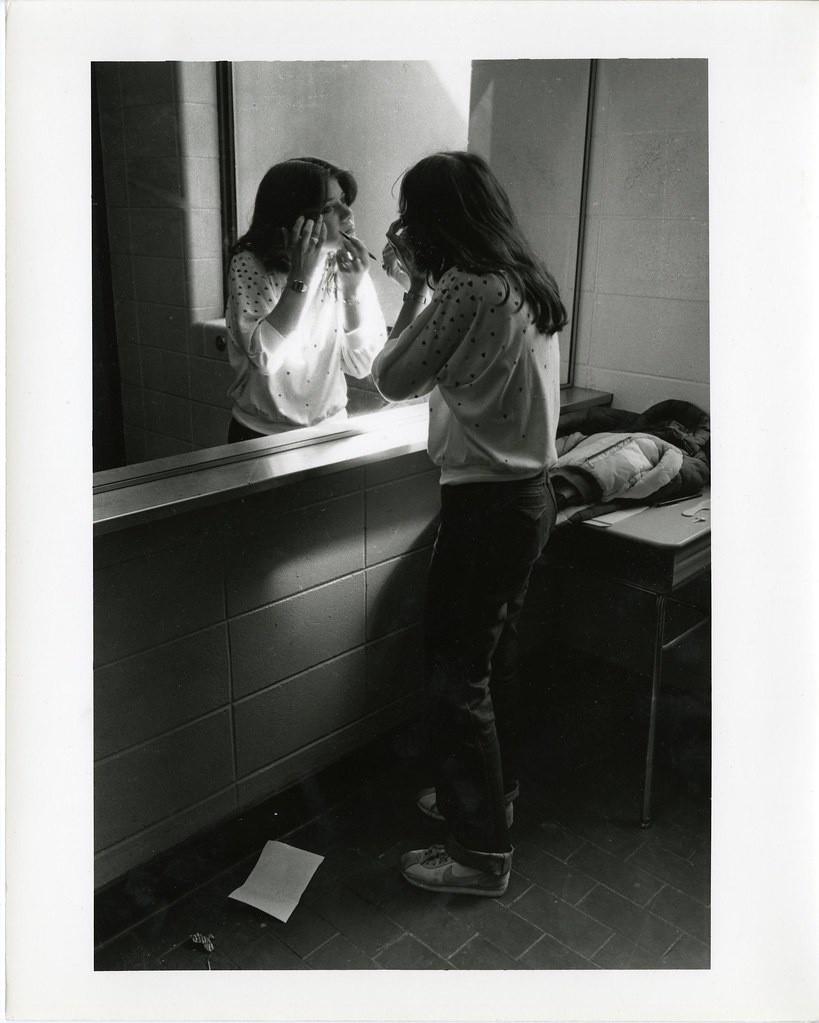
[403,291,427,304]
[286,280,308,295]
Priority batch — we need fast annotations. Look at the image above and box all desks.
[540,485,711,829]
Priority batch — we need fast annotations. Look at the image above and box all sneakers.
[417,787,514,830]
[400,845,510,897]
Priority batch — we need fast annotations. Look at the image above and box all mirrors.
[91,62,599,494]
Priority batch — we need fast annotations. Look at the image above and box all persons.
[371,150,567,898]
[227,157,388,444]
